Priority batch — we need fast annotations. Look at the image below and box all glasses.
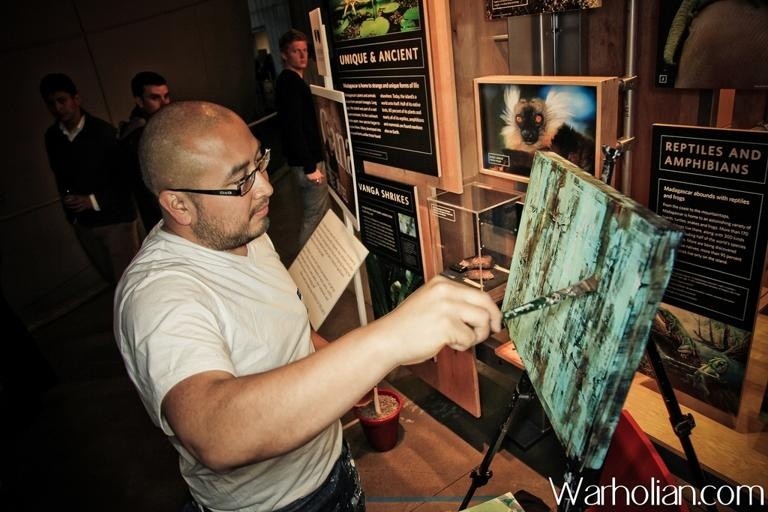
[169,145,273,197]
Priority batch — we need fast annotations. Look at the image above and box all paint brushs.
[502,274,598,320]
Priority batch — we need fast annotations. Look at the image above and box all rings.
[316,179,320,182]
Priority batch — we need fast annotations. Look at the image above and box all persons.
[111,100,503,511]
[275,29,331,251]
[39,72,140,285]
[118,70,172,233]
